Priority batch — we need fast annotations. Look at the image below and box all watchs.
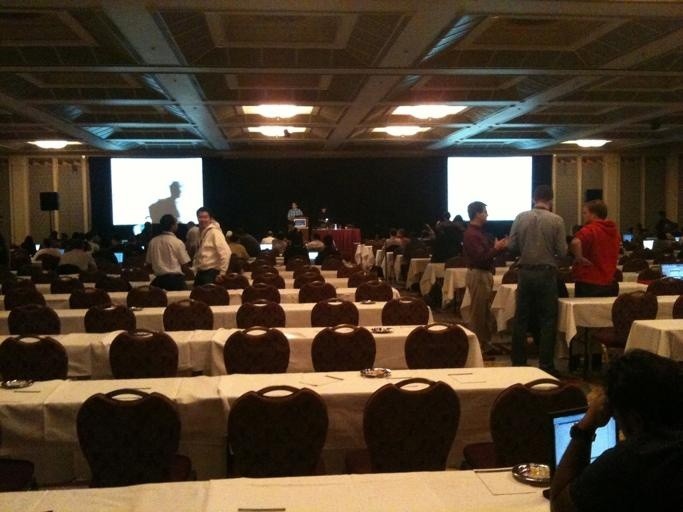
[569,423,596,444]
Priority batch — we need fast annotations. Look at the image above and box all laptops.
[642,239,655,251]
[113,252,124,266]
[260,243,273,252]
[622,232,633,243]
[307,251,319,262]
[35,244,41,251]
[545,405,619,499]
[660,263,683,281]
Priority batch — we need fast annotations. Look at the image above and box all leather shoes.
[483,353,496,361]
[488,347,503,356]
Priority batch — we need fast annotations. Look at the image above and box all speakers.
[39,191,59,212]
[585,188,603,202]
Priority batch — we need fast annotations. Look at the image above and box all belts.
[521,264,552,271]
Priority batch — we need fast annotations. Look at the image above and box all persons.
[463,201,507,361]
[223,225,342,266]
[550,348,683,512]
[194,207,232,288]
[1,222,151,272]
[624,211,683,264]
[366,213,467,283]
[148,183,185,224]
[504,185,574,376]
[317,208,328,228]
[186,221,200,253]
[571,200,621,299]
[288,200,303,232]
[146,215,191,290]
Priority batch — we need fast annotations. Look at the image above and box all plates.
[513,463,551,487]
[373,328,393,333]
[2,379,33,388]
[361,368,391,378]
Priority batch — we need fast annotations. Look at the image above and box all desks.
[311,227,360,250]
